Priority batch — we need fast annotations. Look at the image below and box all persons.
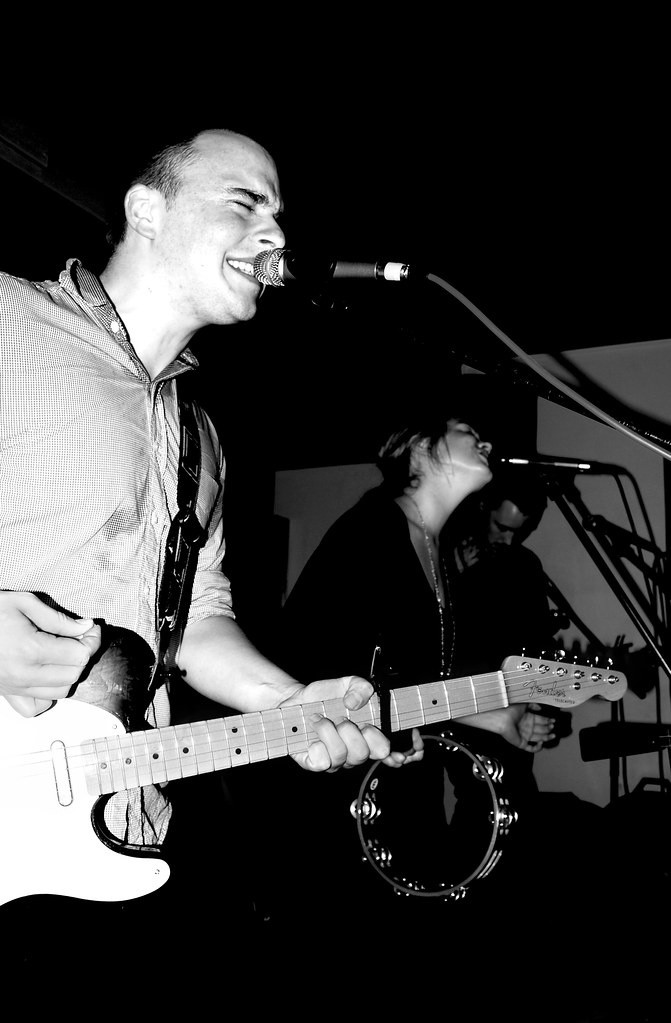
[0,109,391,1023]
[286,405,559,822]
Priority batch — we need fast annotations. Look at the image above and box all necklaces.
[405,488,458,679]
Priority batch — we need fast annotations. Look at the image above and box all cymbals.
[348,729,522,908]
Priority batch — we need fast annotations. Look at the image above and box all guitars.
[0,625,632,911]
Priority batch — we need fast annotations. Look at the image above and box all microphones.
[253,249,429,286]
[583,513,661,556]
[490,447,615,476]
